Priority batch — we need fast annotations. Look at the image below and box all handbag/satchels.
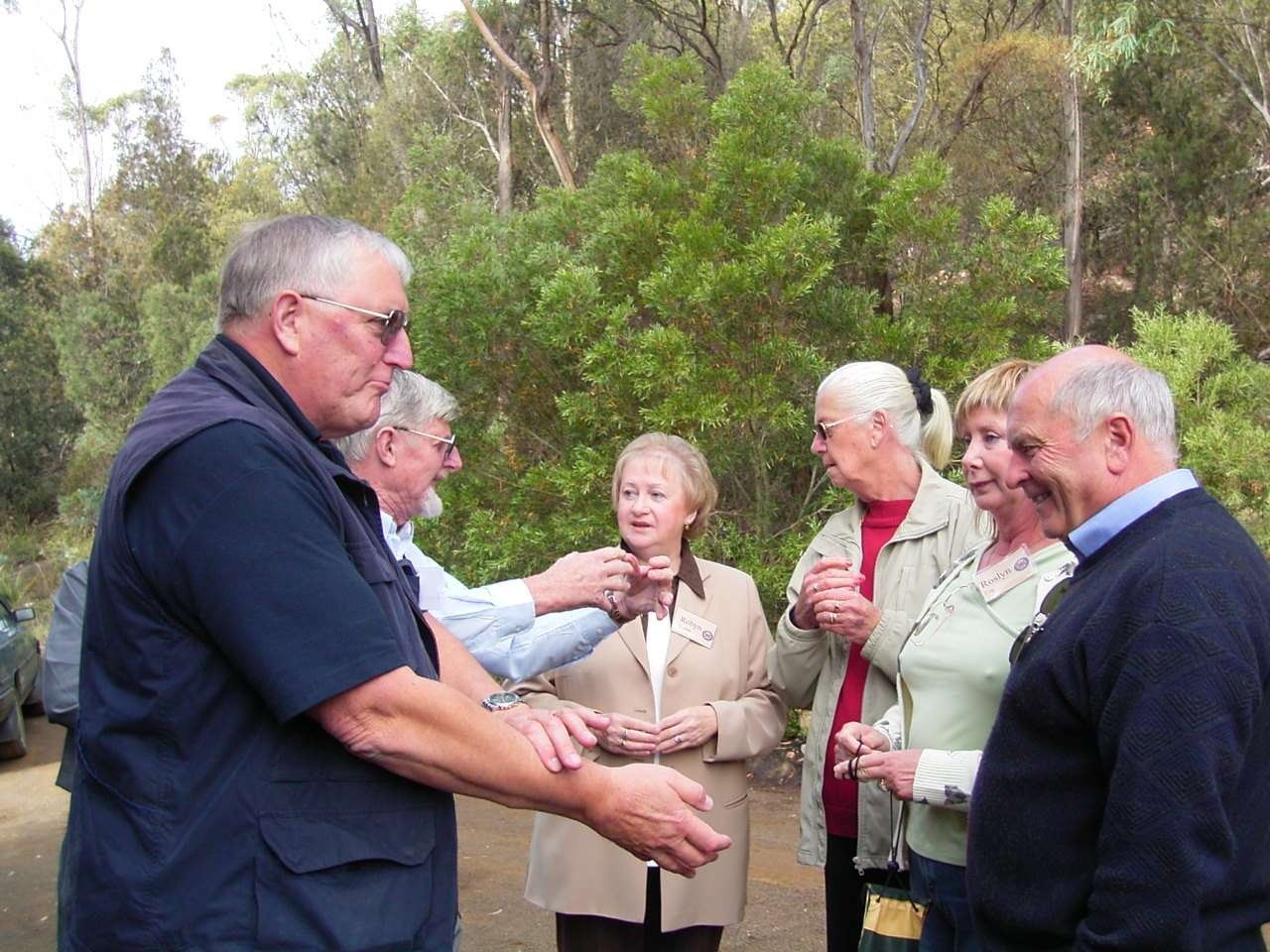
[859,865,929,951]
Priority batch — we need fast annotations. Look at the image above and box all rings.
[879,778,889,794]
[835,599,841,613]
[622,729,627,739]
[830,613,837,625]
[674,734,684,743]
[620,738,626,748]
[813,582,819,593]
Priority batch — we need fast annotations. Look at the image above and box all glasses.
[391,424,457,456]
[269,293,412,347]
[811,412,869,442]
[1010,577,1075,666]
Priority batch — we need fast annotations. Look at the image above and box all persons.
[331,366,674,683]
[833,362,1080,952]
[505,432,788,952]
[964,345,1270,952]
[55,214,731,952]
[41,558,90,793]
[766,362,1000,952]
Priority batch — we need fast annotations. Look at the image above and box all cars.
[0,588,46,761]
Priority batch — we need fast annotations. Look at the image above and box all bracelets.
[606,589,634,626]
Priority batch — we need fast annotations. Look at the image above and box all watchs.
[480,691,533,711]
